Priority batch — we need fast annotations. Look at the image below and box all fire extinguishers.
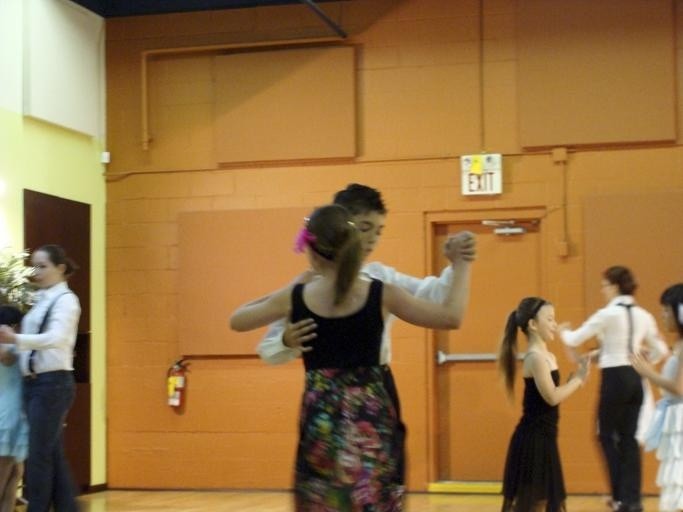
[168,359,188,406]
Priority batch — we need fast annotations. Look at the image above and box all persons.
[556,266,669,512]
[0,304,30,512]
[565,346,656,509]
[498,296,592,512]
[0,244,82,512]
[627,282,683,512]
[256,183,478,419]
[229,205,470,512]
